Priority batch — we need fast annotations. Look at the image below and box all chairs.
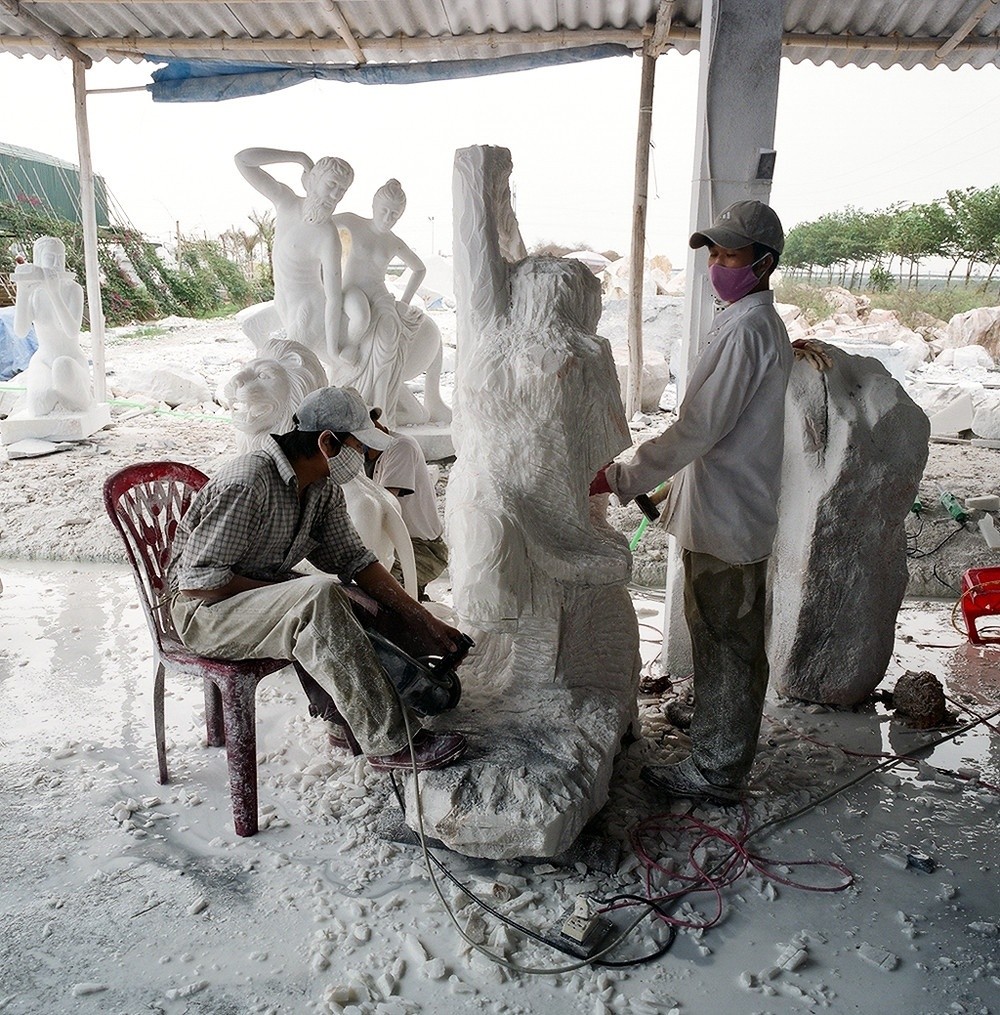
[102,461,363,839]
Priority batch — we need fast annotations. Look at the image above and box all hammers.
[634,482,672,523]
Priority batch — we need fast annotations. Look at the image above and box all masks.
[318,429,365,485]
[709,252,773,301]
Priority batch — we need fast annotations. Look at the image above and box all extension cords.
[561,907,601,944]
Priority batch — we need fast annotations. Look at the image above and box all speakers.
[754,148,777,181]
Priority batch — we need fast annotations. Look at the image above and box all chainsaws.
[364,630,475,718]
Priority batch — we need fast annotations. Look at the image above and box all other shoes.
[641,764,738,806]
[367,728,468,770]
[665,702,695,727]
[328,733,350,748]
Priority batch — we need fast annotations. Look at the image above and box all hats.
[689,200,785,253]
[293,386,394,451]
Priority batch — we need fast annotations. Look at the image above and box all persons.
[231,145,353,364]
[165,387,473,774]
[331,175,428,431]
[585,200,794,807]
[345,404,451,603]
[9,237,95,415]
